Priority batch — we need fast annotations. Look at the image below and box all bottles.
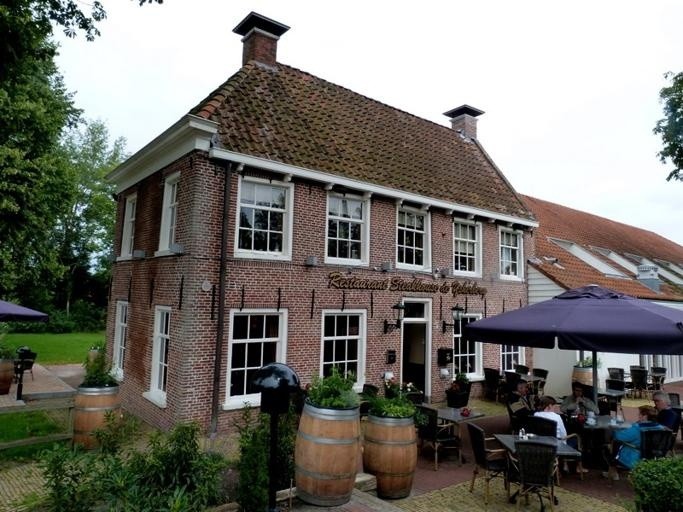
[576,403,581,417]
[519,427,525,438]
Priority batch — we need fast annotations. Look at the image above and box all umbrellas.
[0,298,49,318]
[462,283,683,407]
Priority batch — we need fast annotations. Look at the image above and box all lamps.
[302,254,450,280]
[442,301,464,335]
[133,242,187,260]
[383,299,405,335]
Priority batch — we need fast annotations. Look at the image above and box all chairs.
[466,362,682,511]
[351,383,463,473]
[11,348,38,385]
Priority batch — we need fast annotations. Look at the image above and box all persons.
[602,404,673,481]
[559,382,600,416]
[652,390,678,431]
[508,379,540,418]
[533,396,590,474]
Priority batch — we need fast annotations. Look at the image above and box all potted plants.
[293,364,432,505]
[69,335,126,452]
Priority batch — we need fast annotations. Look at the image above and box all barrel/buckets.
[293,402,361,507]
[0,357,14,395]
[73,386,121,452]
[88,349,98,372]
[363,411,417,500]
[572,367,600,391]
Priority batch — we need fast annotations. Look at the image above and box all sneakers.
[563,464,569,472]
[603,472,620,480]
[576,465,589,473]
